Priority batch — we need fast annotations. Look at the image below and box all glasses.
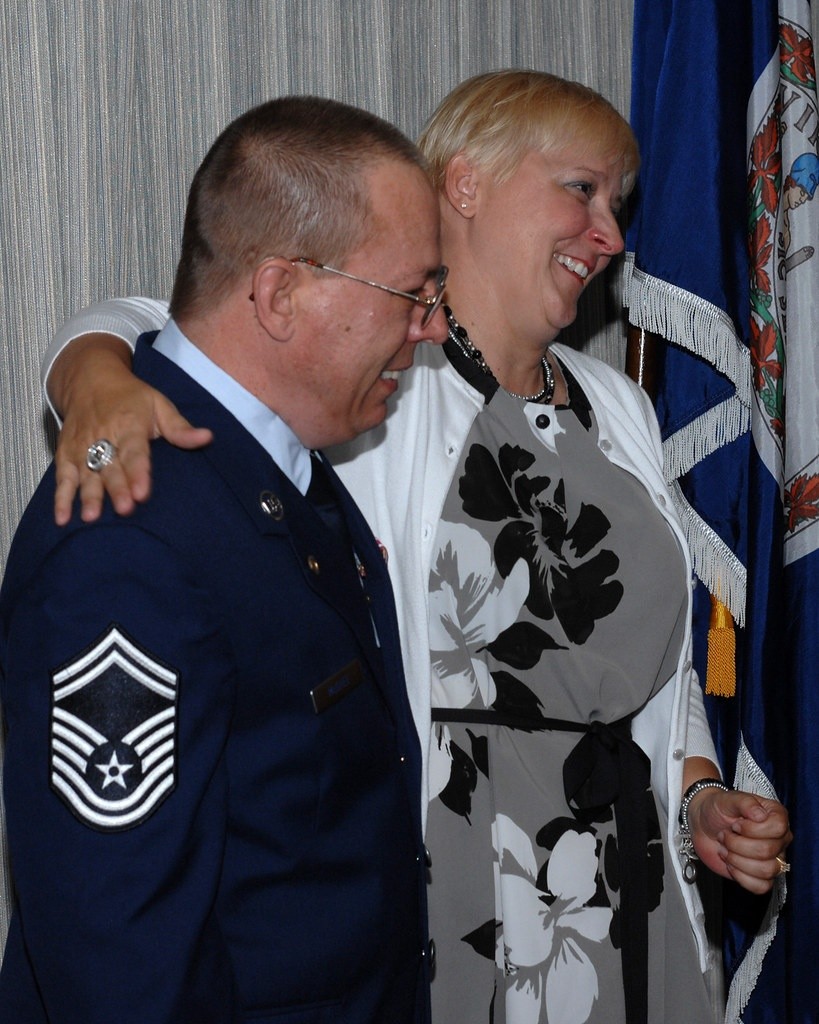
[248,256,450,330]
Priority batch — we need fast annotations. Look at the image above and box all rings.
[87,439,114,470]
[775,856,791,876]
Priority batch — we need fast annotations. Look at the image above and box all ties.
[307,454,358,570]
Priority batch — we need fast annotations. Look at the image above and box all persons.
[0,95,450,1024]
[39,68,793,1024]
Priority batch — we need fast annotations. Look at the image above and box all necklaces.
[444,304,554,404]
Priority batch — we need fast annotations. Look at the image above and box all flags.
[617,0,819,1024]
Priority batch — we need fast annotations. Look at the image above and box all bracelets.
[678,778,729,884]
